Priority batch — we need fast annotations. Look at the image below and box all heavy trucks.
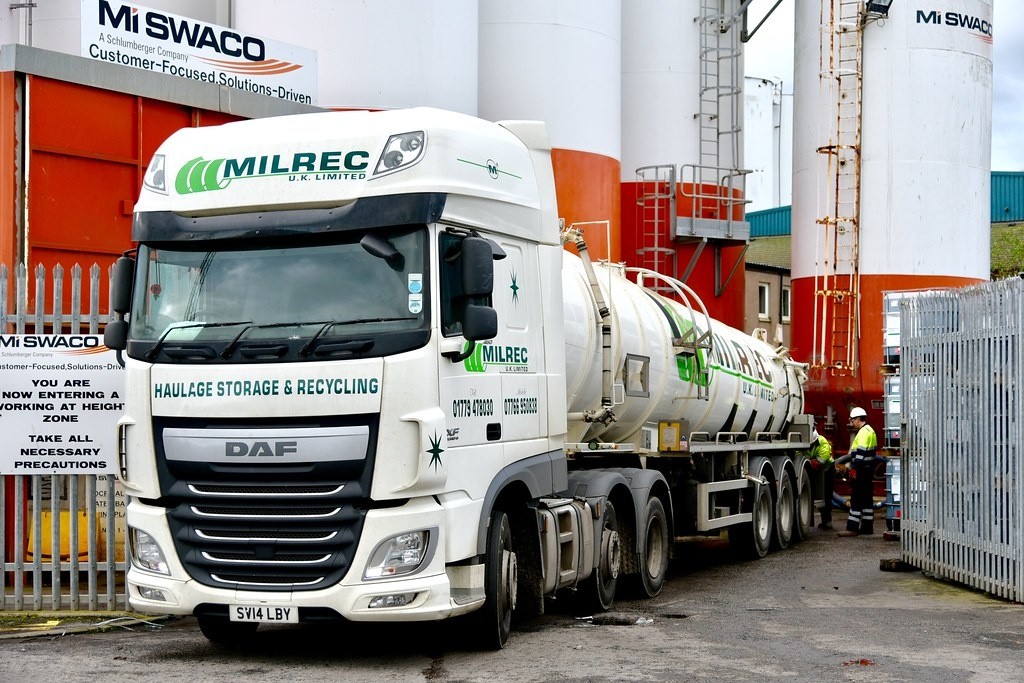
[105,104,815,652]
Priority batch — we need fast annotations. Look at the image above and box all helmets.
[812,429,818,443]
[849,407,867,420]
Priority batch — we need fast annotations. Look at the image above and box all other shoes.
[818,523,833,531]
[837,530,859,537]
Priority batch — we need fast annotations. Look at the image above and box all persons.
[833,407,877,536]
[796,428,836,530]
